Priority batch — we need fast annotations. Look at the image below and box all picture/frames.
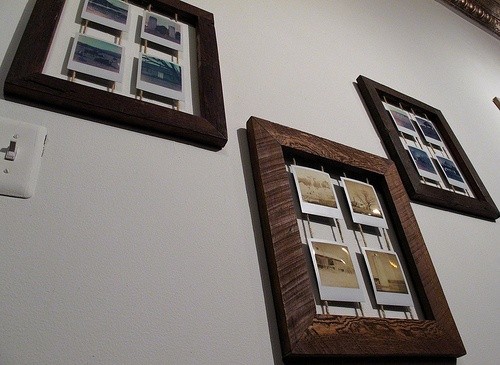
[356,73,500,220]
[3,0,228,154]
[245,113,466,365]
